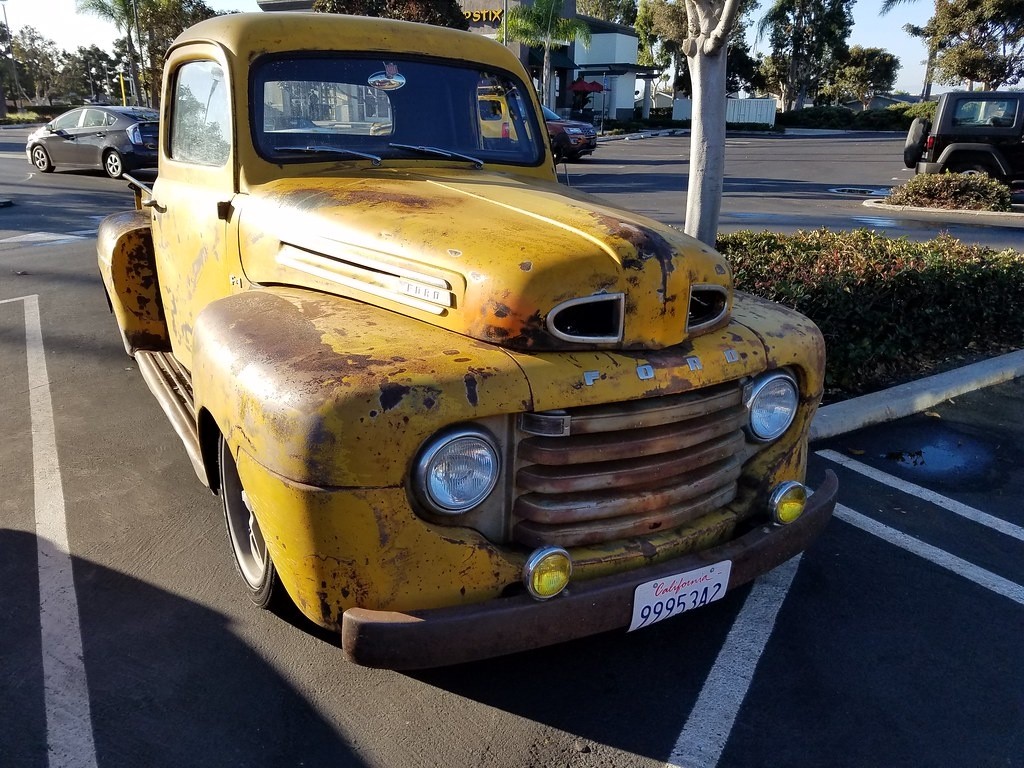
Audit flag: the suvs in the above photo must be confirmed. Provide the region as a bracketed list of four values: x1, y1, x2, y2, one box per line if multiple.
[902, 87, 1024, 186]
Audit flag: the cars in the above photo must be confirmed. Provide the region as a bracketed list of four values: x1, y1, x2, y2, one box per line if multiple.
[542, 104, 598, 164]
[94, 9, 841, 667]
[24, 105, 160, 180]
[369, 84, 532, 143]
[264, 113, 339, 134]
